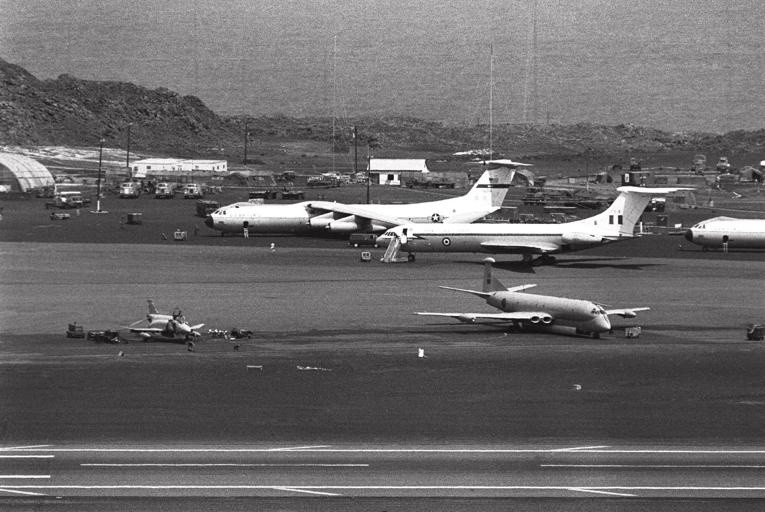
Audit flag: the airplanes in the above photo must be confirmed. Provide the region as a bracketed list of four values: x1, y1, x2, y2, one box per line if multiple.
[120, 298, 205, 343]
[411, 257, 651, 340]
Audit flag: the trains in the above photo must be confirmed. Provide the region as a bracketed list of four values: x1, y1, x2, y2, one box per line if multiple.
[49, 183, 102, 196]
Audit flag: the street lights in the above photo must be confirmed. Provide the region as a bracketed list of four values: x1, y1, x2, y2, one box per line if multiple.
[95, 138, 106, 211]
[127, 122, 133, 168]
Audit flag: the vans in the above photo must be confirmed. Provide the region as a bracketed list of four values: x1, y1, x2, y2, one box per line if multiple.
[45, 191, 84, 209]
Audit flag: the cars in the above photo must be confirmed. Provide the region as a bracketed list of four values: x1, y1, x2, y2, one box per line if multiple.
[155, 183, 175, 199]
[184, 184, 204, 199]
[120, 182, 140, 198]
